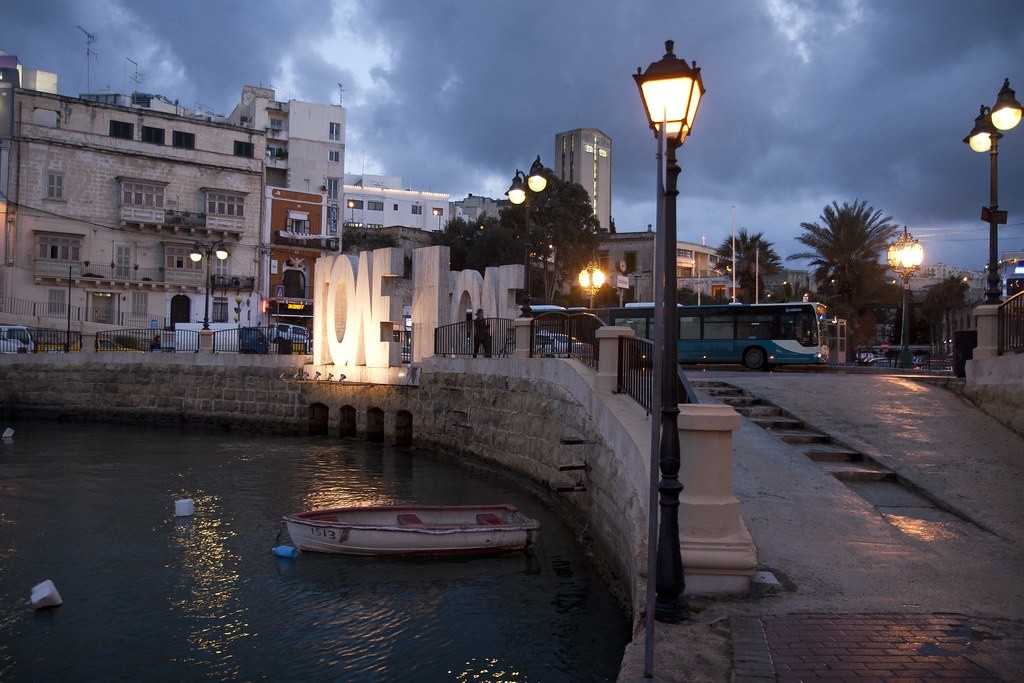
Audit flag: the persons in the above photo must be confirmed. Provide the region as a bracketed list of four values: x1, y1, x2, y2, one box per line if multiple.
[473, 309, 490, 357]
[151, 335, 161, 350]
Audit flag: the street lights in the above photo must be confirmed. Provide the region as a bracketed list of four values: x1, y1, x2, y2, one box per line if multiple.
[434, 211, 440, 230]
[887, 226, 924, 369]
[349, 202, 354, 222]
[579, 261, 605, 309]
[632, 39, 705, 624]
[963, 78, 1024, 304]
[504, 155, 547, 317]
[190, 240, 228, 330]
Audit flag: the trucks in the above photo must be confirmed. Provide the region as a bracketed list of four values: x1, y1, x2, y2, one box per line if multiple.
[175, 322, 270, 355]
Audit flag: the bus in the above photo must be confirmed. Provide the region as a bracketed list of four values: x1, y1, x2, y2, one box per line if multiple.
[610, 302, 830, 370]
[467, 305, 587, 336]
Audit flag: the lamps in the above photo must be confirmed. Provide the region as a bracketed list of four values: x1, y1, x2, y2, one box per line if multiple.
[303, 372, 309, 378]
[327, 373, 333, 381]
[340, 374, 346, 381]
[314, 372, 321, 379]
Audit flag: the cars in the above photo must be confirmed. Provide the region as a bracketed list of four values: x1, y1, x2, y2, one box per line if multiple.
[70, 337, 136, 351]
[270, 323, 310, 344]
[0, 326, 34, 354]
[534, 330, 594, 359]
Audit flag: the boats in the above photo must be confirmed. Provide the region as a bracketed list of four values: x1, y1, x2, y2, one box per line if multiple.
[282, 505, 542, 558]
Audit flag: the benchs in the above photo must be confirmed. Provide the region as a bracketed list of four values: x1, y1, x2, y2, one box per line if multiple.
[317, 517, 339, 521]
[476, 514, 507, 525]
[398, 514, 425, 525]
[498, 335, 556, 357]
